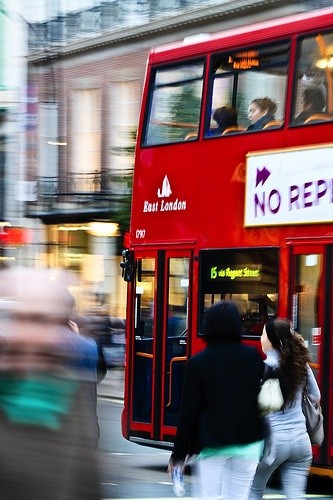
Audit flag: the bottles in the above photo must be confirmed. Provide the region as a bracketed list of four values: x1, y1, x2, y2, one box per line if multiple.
[172, 464, 185, 497]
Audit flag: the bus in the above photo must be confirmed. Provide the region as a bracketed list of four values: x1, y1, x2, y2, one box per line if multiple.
[119, 3, 333, 496]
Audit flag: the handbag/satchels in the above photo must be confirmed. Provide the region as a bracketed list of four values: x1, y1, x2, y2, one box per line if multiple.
[304, 383, 324, 446]
